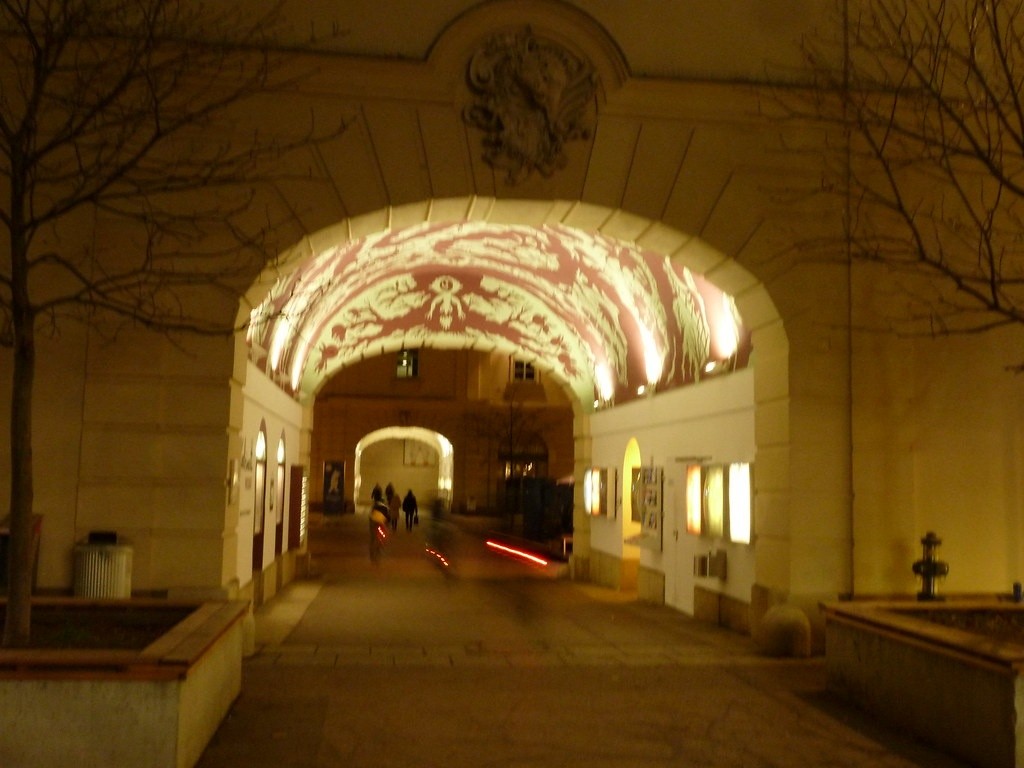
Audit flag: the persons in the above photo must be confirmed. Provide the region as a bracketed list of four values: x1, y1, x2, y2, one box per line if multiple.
[385, 481, 395, 505]
[371, 482, 382, 505]
[402, 490, 418, 533]
[368, 498, 391, 566]
[388, 491, 401, 532]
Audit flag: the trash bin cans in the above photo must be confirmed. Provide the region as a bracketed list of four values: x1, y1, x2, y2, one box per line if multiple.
[72, 543, 134, 598]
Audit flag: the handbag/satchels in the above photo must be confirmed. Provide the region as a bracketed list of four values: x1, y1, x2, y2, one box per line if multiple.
[414, 513, 419, 525]
